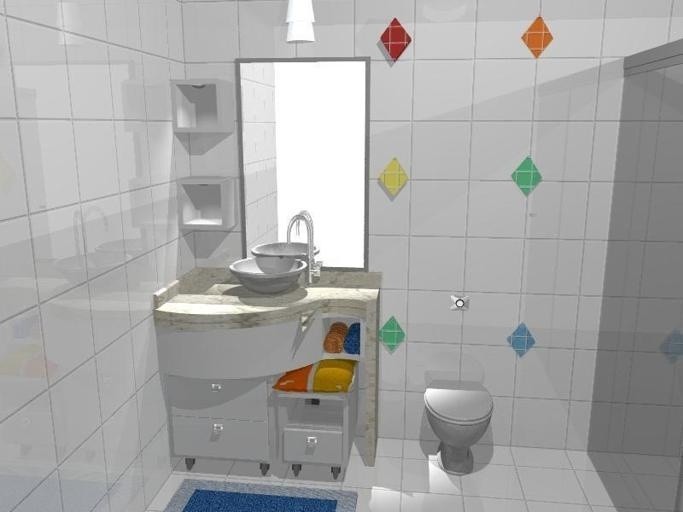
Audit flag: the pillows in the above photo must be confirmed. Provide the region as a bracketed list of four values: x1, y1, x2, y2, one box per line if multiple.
[272, 359, 357, 393]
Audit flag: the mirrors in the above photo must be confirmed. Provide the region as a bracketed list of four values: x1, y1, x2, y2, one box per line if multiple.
[233, 55, 370, 273]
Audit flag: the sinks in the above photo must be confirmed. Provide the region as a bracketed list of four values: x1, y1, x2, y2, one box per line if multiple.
[252, 241, 319, 256]
[229, 256, 308, 294]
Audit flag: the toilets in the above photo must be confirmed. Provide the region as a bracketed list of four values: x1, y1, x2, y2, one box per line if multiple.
[423, 381, 493, 473]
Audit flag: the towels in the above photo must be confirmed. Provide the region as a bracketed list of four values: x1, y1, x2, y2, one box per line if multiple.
[344, 322, 360, 354]
[323, 320, 348, 353]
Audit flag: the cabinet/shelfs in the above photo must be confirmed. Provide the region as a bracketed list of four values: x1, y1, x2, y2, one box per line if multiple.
[158, 314, 362, 480]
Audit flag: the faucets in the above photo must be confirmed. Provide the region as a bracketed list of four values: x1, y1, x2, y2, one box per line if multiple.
[286, 216, 316, 284]
[296, 211, 312, 237]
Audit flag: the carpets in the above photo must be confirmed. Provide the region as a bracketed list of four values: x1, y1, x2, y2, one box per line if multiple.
[164, 478, 358, 512]
